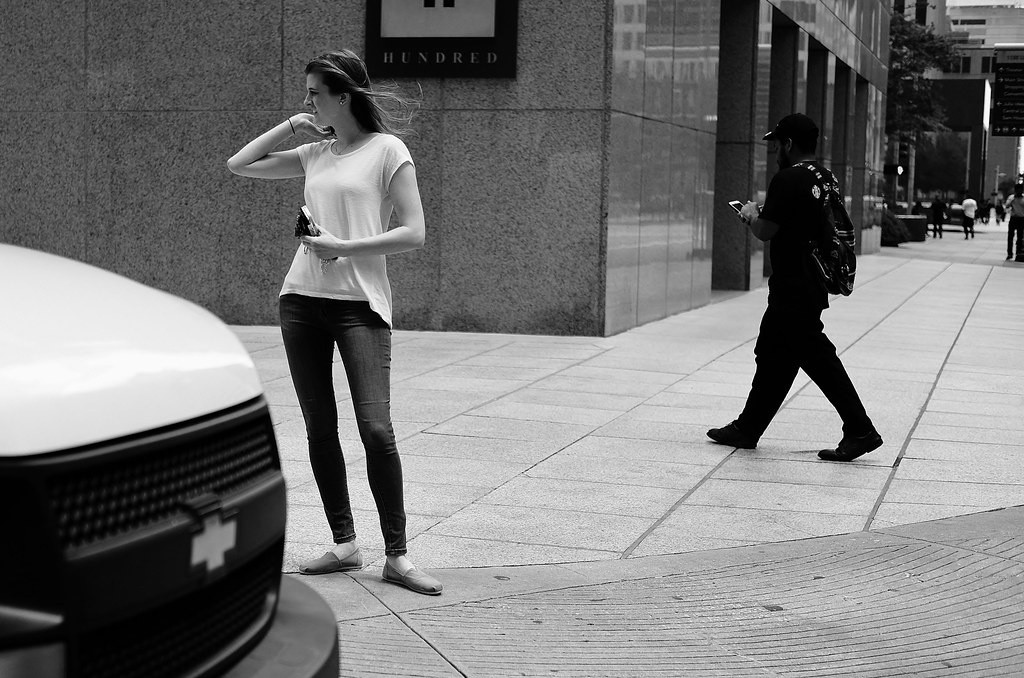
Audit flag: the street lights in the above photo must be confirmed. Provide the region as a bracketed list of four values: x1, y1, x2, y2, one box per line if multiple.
[995, 165, 1006, 209]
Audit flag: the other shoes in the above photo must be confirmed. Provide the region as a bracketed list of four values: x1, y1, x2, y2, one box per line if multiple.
[382, 557, 442, 595]
[818, 428, 882, 461]
[1006, 255, 1013, 260]
[298, 548, 362, 574]
[707, 420, 757, 449]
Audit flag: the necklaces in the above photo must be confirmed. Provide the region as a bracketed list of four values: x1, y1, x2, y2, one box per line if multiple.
[336, 129, 361, 154]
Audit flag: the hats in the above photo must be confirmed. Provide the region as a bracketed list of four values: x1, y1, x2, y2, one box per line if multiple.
[762, 112, 820, 145]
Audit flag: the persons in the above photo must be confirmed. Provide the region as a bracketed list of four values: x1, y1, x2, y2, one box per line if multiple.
[1004, 183, 1024, 263]
[226, 47, 445, 598]
[705, 110, 886, 461]
[912, 188, 1005, 240]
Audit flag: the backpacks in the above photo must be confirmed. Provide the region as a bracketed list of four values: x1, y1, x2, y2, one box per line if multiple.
[793, 161, 856, 297]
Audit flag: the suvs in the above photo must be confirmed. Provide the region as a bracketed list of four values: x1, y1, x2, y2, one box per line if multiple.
[0, 246, 339, 678]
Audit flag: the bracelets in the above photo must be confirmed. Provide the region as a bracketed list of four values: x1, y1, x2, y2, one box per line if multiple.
[287, 117, 297, 136]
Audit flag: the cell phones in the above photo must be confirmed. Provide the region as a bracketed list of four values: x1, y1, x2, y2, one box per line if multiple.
[302, 205, 320, 236]
[729, 200, 744, 216]
